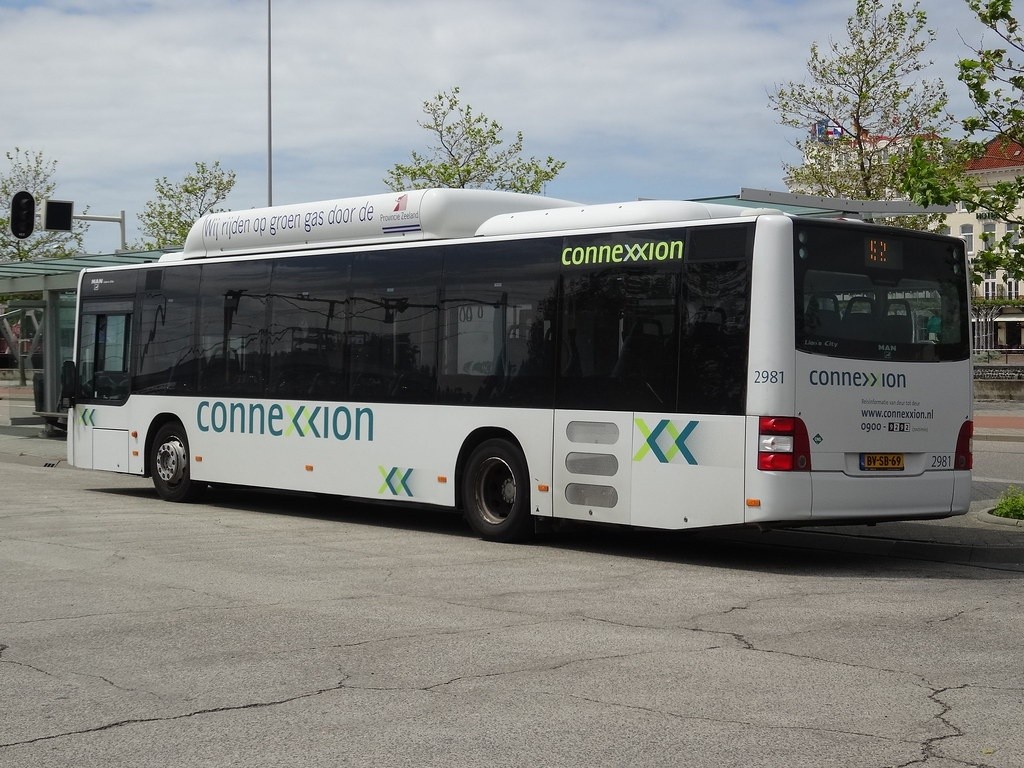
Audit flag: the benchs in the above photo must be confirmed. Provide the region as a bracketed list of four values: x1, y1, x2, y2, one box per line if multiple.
[32, 392, 68, 436]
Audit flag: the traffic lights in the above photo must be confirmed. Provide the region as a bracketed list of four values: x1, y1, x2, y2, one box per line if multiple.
[43, 197, 74, 232]
[10, 188, 36, 238]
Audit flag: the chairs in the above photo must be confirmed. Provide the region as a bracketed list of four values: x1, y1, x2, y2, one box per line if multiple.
[173, 293, 915, 402]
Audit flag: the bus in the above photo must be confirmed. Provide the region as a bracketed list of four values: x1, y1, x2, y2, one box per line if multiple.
[56, 174, 976, 539]
